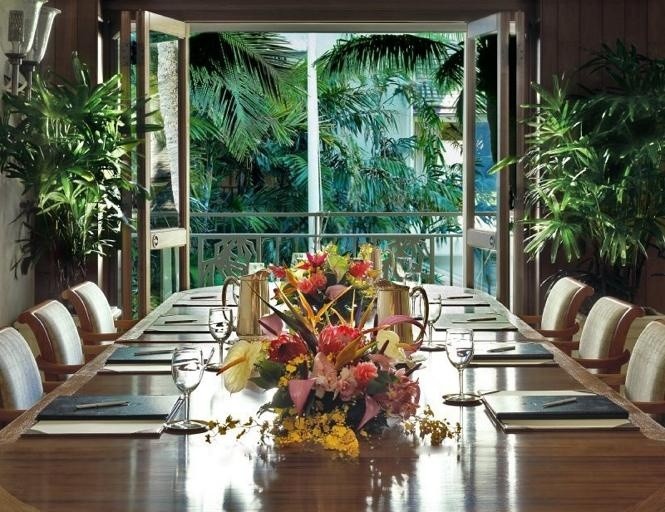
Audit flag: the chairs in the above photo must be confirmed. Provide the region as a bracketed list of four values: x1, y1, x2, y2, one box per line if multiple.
[0, 277, 141, 432]
[519, 277, 664, 424]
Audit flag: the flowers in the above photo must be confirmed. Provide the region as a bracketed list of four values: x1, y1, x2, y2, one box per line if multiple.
[201, 243, 464, 462]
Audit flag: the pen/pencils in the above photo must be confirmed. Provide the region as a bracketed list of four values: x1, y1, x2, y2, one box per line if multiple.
[543, 398, 577, 408]
[75, 400, 129, 410]
[190, 296, 216, 299]
[447, 295, 473, 299]
[134, 350, 173, 355]
[487, 346, 515, 352]
[467, 318, 496, 321]
[165, 320, 198, 324]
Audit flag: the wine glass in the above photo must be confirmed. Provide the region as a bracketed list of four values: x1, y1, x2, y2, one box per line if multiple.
[207, 307, 236, 371]
[443, 326, 482, 404]
[394, 255, 423, 318]
[418, 292, 445, 351]
[162, 346, 212, 432]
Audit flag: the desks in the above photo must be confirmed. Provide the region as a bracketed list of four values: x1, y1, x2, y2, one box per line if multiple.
[1, 282, 664, 512]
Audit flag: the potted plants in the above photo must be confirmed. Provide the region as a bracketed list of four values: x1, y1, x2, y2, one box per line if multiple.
[0, 50, 164, 397]
[487, 38, 665, 396]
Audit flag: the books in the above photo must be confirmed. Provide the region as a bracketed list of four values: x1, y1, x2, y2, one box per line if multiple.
[18, 290, 242, 439]
[420, 292, 643, 432]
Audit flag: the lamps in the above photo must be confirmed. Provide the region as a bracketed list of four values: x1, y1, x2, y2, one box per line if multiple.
[0, 0, 63, 101]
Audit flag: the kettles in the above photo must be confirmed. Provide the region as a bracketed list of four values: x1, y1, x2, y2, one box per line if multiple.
[372, 280, 430, 359]
[221, 268, 272, 339]
